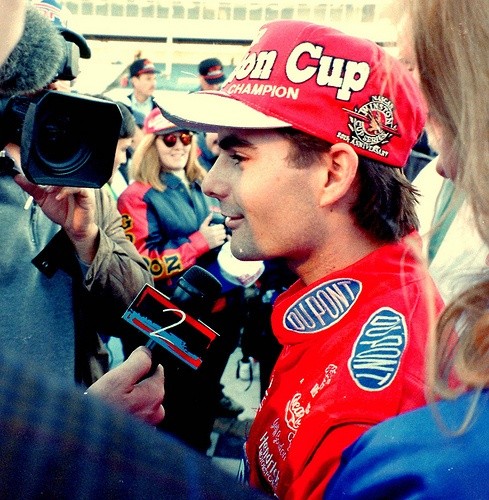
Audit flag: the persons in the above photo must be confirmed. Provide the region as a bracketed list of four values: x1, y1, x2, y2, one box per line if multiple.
[151, 19, 471, 500]
[0, 0, 489, 500]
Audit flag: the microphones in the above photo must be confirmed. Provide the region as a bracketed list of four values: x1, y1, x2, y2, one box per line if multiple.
[1, 3, 66, 96]
[134, 265, 223, 386]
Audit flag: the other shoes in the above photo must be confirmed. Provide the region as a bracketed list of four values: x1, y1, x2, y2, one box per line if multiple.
[218, 398, 244, 416]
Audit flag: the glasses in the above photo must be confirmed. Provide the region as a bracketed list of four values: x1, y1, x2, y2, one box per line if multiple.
[158, 132, 194, 148]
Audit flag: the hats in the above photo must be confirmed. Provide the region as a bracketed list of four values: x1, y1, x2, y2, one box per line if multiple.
[145, 108, 200, 136]
[129, 59, 160, 76]
[199, 57, 226, 85]
[152, 19, 427, 167]
